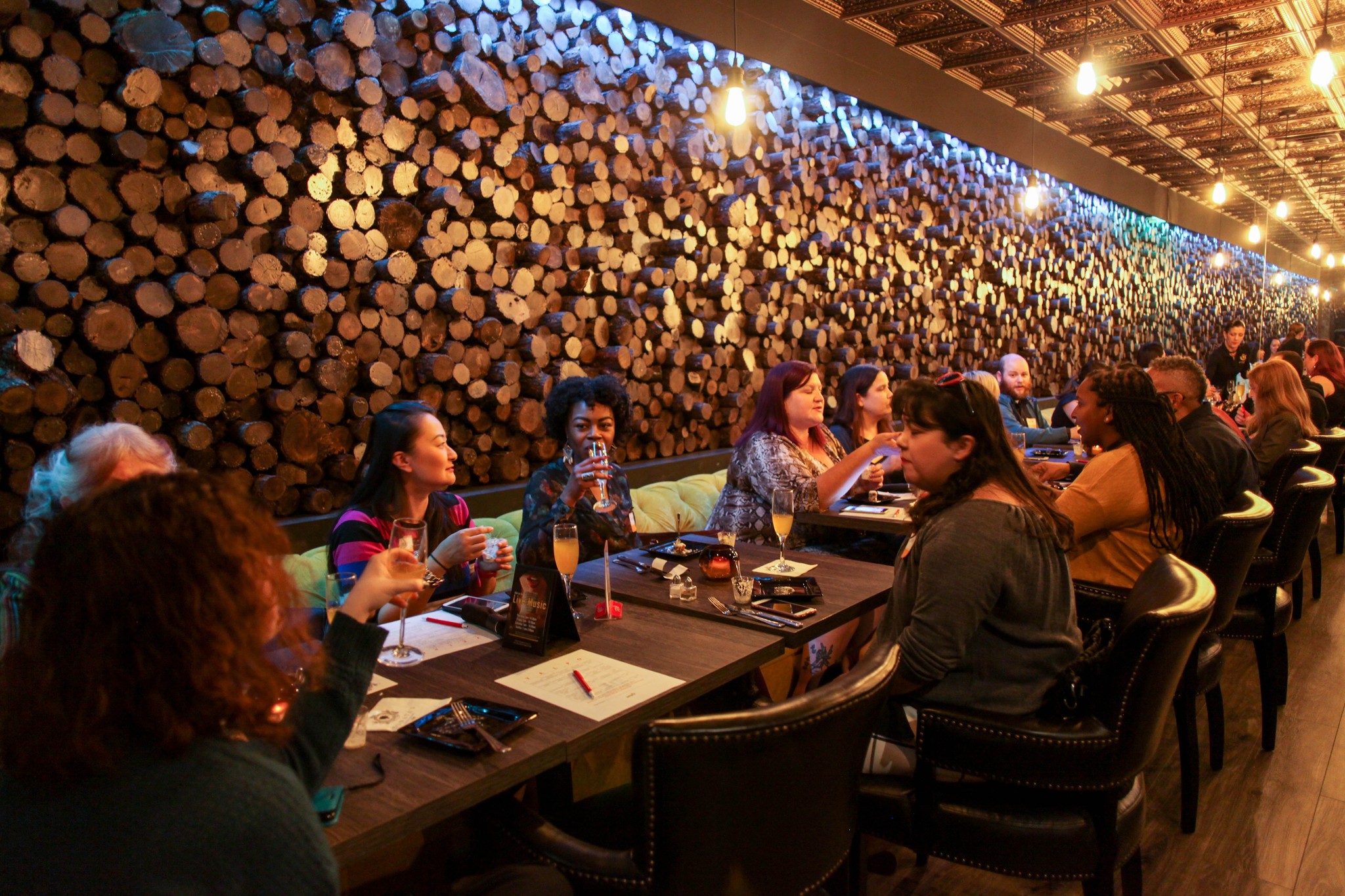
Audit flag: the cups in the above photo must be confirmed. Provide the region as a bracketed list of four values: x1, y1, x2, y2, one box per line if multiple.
[1091, 446, 1103, 458]
[1011, 433, 1025, 463]
[344, 705, 369, 749]
[326, 572, 357, 625]
[731, 576, 754, 604]
[1073, 443, 1084, 457]
[717, 531, 737, 547]
[482, 538, 498, 563]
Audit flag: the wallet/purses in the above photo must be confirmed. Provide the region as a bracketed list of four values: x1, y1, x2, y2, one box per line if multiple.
[309, 753, 386, 826]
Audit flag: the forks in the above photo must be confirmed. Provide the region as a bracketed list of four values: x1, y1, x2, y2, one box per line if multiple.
[707, 596, 785, 627]
[451, 702, 512, 753]
[612, 559, 653, 574]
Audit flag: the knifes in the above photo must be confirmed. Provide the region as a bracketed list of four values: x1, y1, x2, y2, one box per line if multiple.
[711, 603, 803, 629]
[617, 555, 673, 577]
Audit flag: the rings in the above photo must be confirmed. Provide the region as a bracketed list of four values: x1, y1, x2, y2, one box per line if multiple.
[580, 472, 594, 481]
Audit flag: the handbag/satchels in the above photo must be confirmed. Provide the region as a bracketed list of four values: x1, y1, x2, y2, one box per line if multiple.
[1037, 617, 1119, 727]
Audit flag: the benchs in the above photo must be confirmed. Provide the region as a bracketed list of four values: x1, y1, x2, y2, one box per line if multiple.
[276, 453, 744, 617]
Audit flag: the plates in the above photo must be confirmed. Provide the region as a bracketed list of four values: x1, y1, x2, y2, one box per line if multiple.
[840, 492, 901, 506]
[639, 539, 710, 557]
[1030, 449, 1068, 457]
[397, 696, 538, 757]
[753, 577, 823, 601]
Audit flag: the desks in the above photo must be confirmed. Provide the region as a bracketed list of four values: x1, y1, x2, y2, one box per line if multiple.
[800, 492, 917, 538]
[249, 538, 897, 890]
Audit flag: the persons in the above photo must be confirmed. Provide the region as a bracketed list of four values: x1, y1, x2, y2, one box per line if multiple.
[704, 320, 1345, 583]
[0, 424, 423, 896]
[863, 372, 1081, 877]
[512, 570, 548, 631]
[328, 401, 517, 629]
[515, 375, 659, 568]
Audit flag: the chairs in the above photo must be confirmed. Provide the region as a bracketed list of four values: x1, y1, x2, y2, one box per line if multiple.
[489, 641, 900, 896]
[862, 554, 1215, 896]
[1261, 427, 1345, 619]
[1173, 491, 1274, 829]
[1217, 465, 1336, 748]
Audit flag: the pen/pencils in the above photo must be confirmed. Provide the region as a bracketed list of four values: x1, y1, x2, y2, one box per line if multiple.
[573, 670, 595, 698]
[1075, 459, 1087, 463]
[426, 616, 467, 627]
[893, 509, 900, 517]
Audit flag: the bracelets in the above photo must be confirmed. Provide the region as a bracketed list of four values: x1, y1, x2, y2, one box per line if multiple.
[422, 554, 447, 587]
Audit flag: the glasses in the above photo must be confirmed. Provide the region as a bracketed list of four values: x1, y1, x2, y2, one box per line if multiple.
[936, 372, 983, 429]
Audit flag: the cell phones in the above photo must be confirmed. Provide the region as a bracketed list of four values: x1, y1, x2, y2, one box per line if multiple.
[749, 598, 817, 619]
[309, 785, 345, 827]
[442, 595, 510, 615]
[676, 534, 721, 545]
[839, 505, 889, 513]
[1023, 455, 1048, 461]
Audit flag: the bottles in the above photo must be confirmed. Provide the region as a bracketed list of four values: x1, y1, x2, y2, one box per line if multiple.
[669, 574, 684, 598]
[699, 544, 739, 582]
[680, 576, 697, 602]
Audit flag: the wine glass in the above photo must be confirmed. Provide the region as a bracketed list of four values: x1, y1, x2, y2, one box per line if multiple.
[769, 488, 796, 572]
[377, 518, 428, 667]
[553, 523, 584, 620]
[588, 441, 617, 513]
[1207, 380, 1245, 405]
